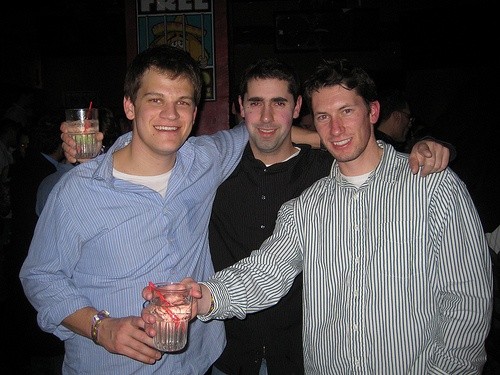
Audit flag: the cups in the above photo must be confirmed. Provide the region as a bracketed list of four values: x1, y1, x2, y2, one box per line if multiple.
[65, 108, 99, 163]
[151, 282, 192, 352]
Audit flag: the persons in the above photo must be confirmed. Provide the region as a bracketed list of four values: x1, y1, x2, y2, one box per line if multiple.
[19, 45, 327, 375]
[232, 93, 415, 153]
[61, 58, 449, 375]
[142, 65, 493, 375]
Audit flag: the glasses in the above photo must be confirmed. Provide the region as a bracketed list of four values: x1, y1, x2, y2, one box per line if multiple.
[393, 109, 415, 122]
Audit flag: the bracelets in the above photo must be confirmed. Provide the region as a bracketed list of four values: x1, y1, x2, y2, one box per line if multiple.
[204, 294, 214, 316]
[92, 310, 111, 344]
